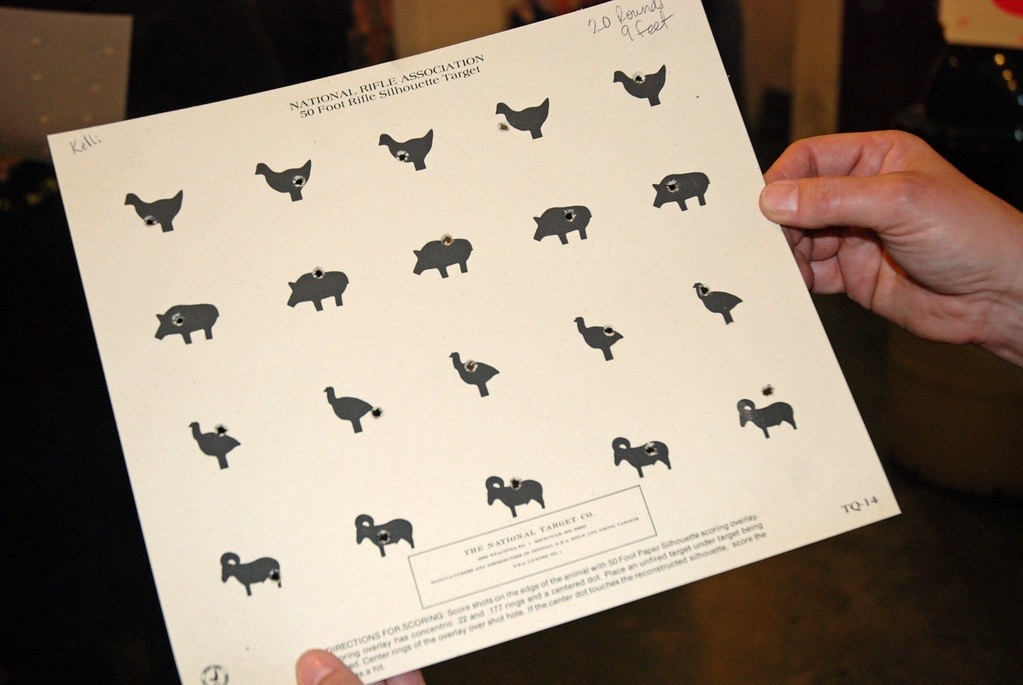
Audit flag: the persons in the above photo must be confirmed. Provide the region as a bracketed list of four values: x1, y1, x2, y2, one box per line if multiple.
[294, 129, 1022, 685]
